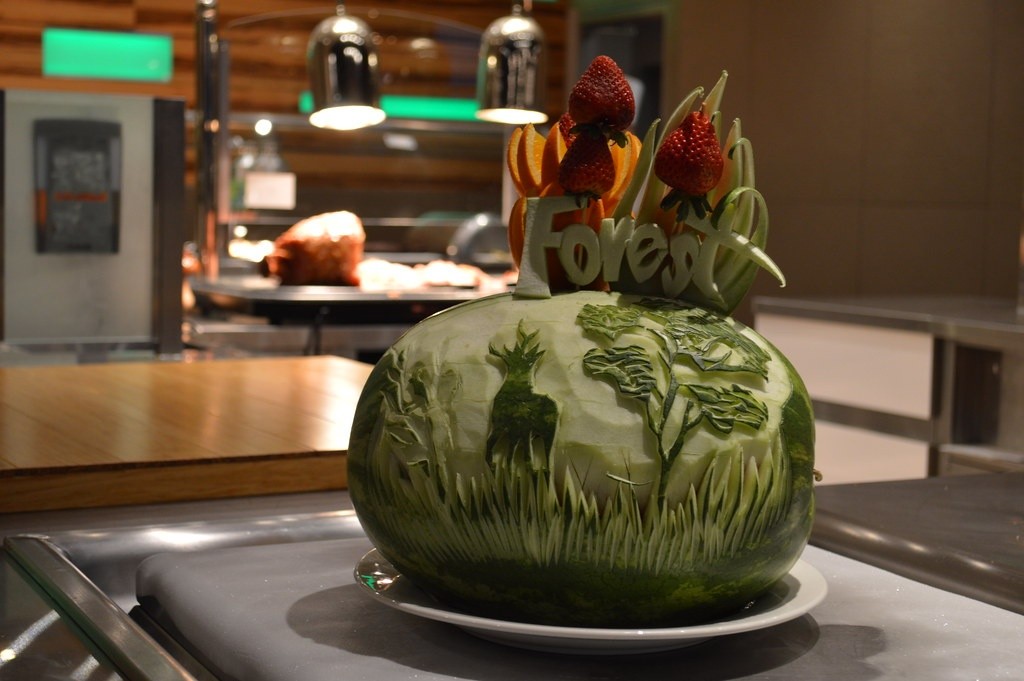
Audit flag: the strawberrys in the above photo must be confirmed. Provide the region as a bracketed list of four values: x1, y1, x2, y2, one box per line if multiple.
[558, 55, 724, 219]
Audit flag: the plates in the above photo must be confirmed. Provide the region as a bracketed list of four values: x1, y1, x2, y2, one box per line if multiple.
[353, 545, 828, 654]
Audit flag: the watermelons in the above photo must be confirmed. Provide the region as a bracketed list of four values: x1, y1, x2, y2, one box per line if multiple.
[346, 288, 817, 618]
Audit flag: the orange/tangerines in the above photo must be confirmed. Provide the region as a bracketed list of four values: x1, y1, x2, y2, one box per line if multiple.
[506, 123, 639, 278]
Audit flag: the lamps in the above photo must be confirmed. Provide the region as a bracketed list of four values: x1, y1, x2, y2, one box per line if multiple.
[308, 11, 390, 134]
[474, 3, 554, 127]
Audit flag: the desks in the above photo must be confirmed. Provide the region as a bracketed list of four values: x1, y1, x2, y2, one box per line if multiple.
[747, 296, 1024, 486]
[1, 352, 376, 518]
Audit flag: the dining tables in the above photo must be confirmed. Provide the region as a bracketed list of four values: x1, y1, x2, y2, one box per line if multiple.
[3, 473, 1022, 681]
